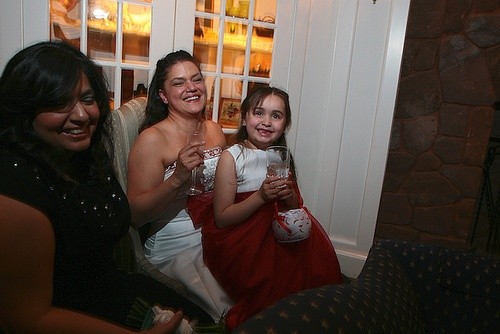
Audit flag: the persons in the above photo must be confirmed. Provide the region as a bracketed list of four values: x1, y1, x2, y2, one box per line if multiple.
[187, 86, 343, 333]
[126, 50, 239, 324]
[0, 40, 197, 334]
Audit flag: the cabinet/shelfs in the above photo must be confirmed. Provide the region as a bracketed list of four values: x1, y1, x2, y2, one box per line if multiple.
[50, 0, 278, 135]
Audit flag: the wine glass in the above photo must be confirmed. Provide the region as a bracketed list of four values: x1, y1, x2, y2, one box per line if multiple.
[183, 131, 204, 196]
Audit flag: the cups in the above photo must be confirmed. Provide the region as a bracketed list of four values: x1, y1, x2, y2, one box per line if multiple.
[265, 145, 290, 190]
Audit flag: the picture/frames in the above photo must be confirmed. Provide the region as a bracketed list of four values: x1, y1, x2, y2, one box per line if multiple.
[220, 98, 240, 128]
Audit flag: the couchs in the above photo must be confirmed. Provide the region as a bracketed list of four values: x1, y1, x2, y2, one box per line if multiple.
[231, 239, 500, 334]
[106, 97, 228, 325]
